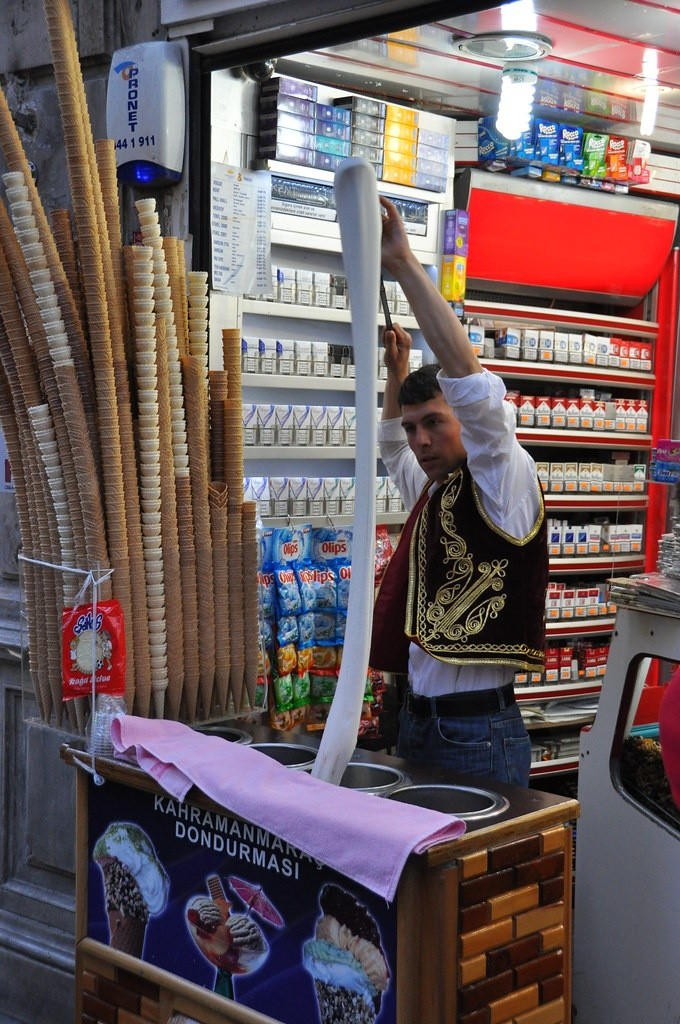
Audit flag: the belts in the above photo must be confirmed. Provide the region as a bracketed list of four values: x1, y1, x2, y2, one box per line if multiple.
[405, 687, 517, 717]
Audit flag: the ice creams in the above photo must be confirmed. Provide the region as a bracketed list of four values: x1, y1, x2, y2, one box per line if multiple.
[303, 883, 392, 1024]
[184, 874, 268, 976]
[92, 822, 171, 961]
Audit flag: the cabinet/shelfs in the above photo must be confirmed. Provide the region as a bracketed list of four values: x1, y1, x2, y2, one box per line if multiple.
[191, 60, 454, 528]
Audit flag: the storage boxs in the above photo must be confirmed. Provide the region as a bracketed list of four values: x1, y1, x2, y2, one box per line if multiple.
[240, 76, 653, 763]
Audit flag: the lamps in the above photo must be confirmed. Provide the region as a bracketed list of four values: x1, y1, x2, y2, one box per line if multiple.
[452, 0, 553, 61]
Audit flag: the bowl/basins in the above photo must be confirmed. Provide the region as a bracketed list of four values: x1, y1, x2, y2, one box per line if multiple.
[91, 695, 126, 760]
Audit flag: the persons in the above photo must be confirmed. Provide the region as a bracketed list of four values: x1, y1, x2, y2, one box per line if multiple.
[369, 194, 547, 792]
